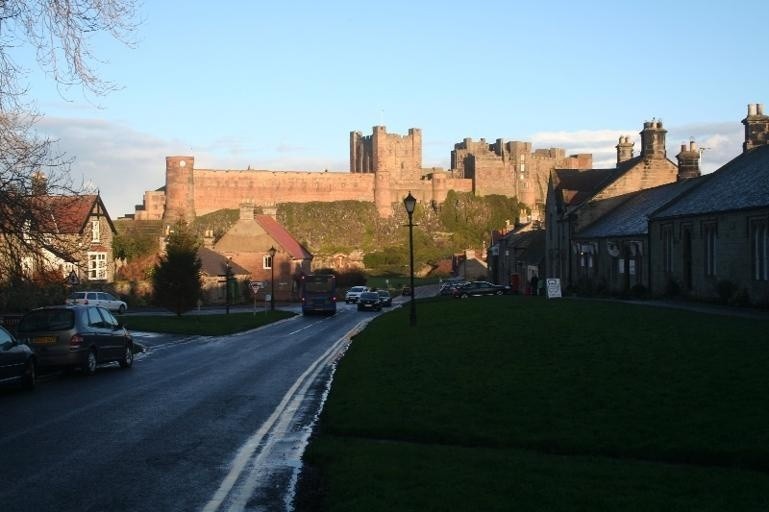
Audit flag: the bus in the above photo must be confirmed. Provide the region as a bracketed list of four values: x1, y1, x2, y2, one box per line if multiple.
[302, 274, 337, 316]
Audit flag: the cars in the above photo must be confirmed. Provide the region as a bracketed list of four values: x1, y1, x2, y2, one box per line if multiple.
[378, 291, 393, 306]
[0, 325, 37, 392]
[357, 292, 382, 311]
[345, 286, 370, 303]
[66, 291, 128, 314]
[402, 287, 411, 296]
[440, 278, 513, 299]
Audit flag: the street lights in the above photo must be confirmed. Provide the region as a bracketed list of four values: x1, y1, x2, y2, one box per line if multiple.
[404, 191, 418, 326]
[225, 259, 229, 314]
[268, 245, 276, 311]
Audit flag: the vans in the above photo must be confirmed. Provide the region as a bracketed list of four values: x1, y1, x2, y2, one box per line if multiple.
[17, 304, 134, 378]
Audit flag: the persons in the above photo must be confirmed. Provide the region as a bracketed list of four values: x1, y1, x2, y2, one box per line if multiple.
[530, 273, 539, 297]
